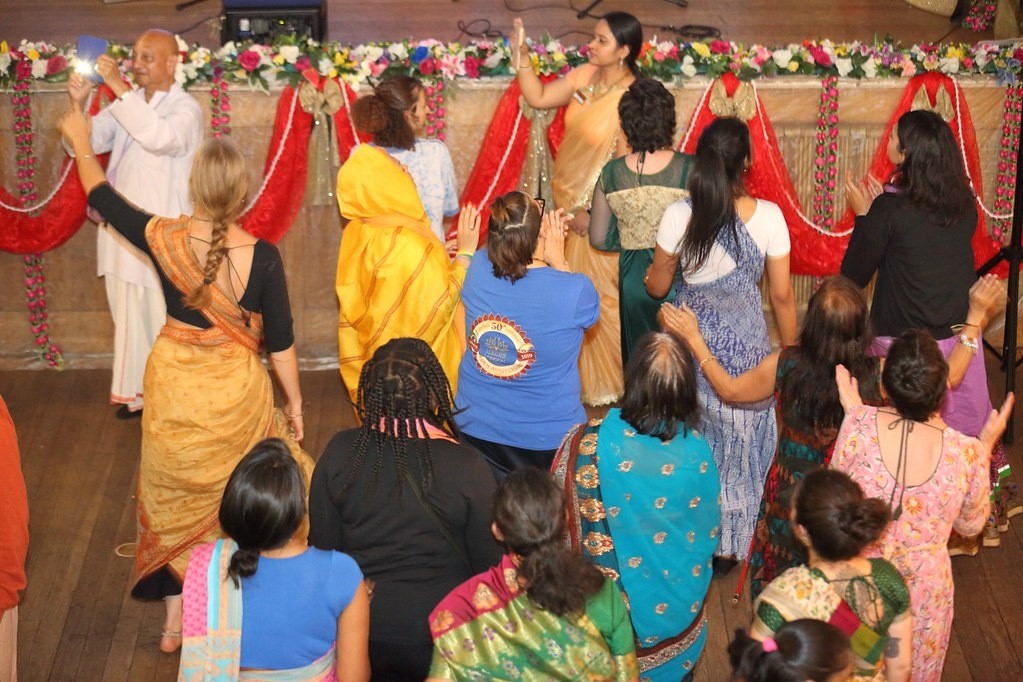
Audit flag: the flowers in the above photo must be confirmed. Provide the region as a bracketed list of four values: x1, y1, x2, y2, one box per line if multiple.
[0, 31, 1023, 373]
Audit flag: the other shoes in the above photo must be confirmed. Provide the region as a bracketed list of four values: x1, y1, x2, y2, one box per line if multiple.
[116, 404, 143, 419]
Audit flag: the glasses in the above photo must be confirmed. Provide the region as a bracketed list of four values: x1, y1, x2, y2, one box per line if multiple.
[534, 197, 546, 221]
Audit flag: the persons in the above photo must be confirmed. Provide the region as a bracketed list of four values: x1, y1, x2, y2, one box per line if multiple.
[424, 465, 639, 682]
[544, 330, 722, 682]
[727, 467, 912, 682]
[178, 438, 371, 682]
[62, 28, 204, 419]
[659, 275, 1005, 626]
[840, 108, 1023, 555]
[307, 337, 510, 682]
[58, 100, 315, 654]
[830, 328, 1013, 682]
[0, 396, 29, 682]
[337, 9, 795, 570]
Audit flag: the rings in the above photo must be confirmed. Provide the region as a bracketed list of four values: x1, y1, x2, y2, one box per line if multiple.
[469, 225, 474, 230]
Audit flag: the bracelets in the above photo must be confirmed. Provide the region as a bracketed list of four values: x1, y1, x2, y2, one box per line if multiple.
[949, 315, 985, 354]
[519, 60, 534, 71]
[76, 155, 96, 161]
[645, 276, 650, 282]
[118, 88, 131, 101]
[698, 355, 719, 371]
[282, 408, 306, 418]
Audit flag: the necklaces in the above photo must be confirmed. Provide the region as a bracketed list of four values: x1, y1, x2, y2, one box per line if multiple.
[532, 255, 546, 265]
[585, 71, 629, 103]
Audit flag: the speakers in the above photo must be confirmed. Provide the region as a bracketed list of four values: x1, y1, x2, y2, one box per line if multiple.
[222, 0, 327, 48]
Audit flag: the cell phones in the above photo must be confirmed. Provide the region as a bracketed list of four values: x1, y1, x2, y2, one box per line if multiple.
[75, 36, 109, 81]
